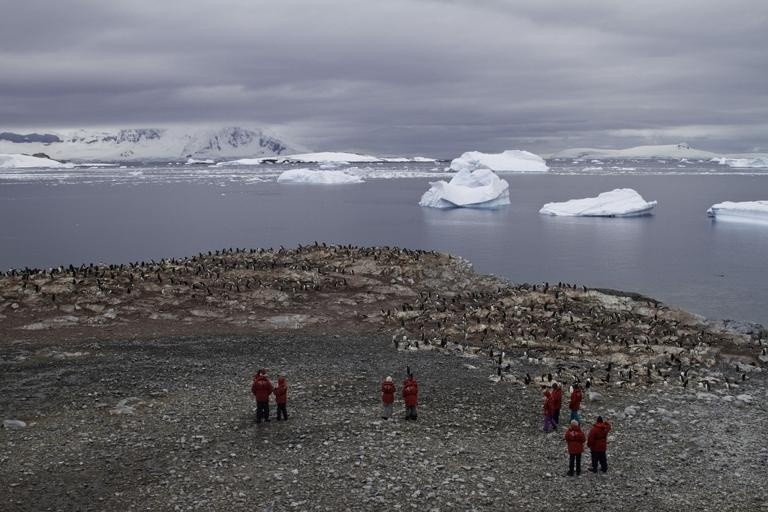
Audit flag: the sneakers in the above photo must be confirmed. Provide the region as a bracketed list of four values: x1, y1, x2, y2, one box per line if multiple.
[276, 415, 289, 421]
[567, 471, 581, 476]
[587, 467, 607, 473]
[405, 415, 418, 420]
[256, 418, 272, 424]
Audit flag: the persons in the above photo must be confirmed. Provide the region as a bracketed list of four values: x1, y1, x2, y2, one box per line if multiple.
[564, 419, 586, 477]
[568, 384, 583, 428]
[253, 368, 266, 417]
[401, 378, 418, 421]
[381, 376, 396, 420]
[273, 375, 289, 420]
[403, 373, 419, 408]
[251, 372, 273, 423]
[586, 415, 612, 473]
[550, 382, 562, 427]
[542, 389, 558, 432]
